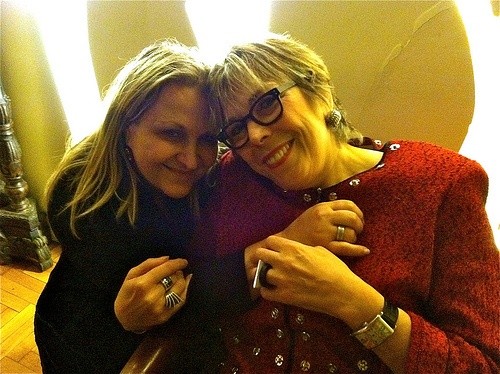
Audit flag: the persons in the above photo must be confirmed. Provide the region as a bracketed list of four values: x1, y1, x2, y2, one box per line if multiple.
[34, 38, 223, 374]
[189, 29, 500, 374]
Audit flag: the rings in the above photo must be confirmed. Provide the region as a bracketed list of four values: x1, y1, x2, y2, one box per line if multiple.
[253, 260, 274, 289]
[161, 276, 172, 289]
[336, 225, 344, 241]
[165, 291, 181, 307]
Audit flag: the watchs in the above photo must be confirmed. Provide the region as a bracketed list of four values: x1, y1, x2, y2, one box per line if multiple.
[352, 297, 399, 350]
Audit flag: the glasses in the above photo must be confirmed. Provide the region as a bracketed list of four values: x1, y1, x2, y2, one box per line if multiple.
[216, 80, 297, 148]
